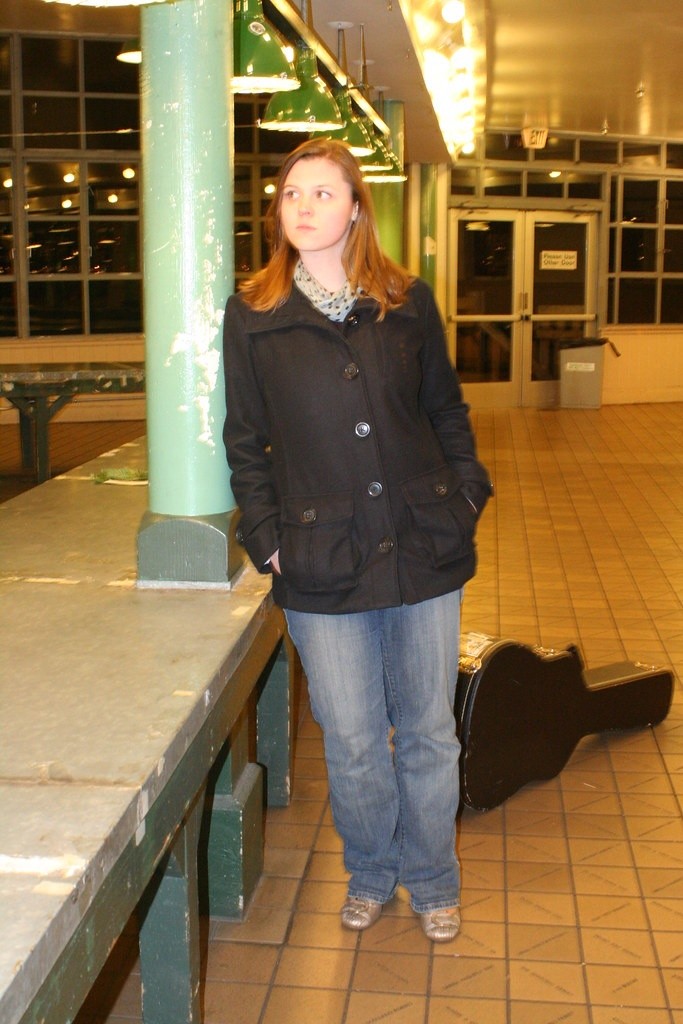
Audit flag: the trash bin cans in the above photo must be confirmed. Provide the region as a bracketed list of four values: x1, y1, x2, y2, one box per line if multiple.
[555, 336, 606, 409]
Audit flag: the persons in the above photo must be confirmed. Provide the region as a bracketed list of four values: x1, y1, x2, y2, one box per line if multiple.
[221, 137, 494, 943]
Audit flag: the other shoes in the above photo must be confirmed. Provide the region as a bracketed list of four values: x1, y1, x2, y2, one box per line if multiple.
[420, 906, 460, 942]
[340, 894, 381, 930]
